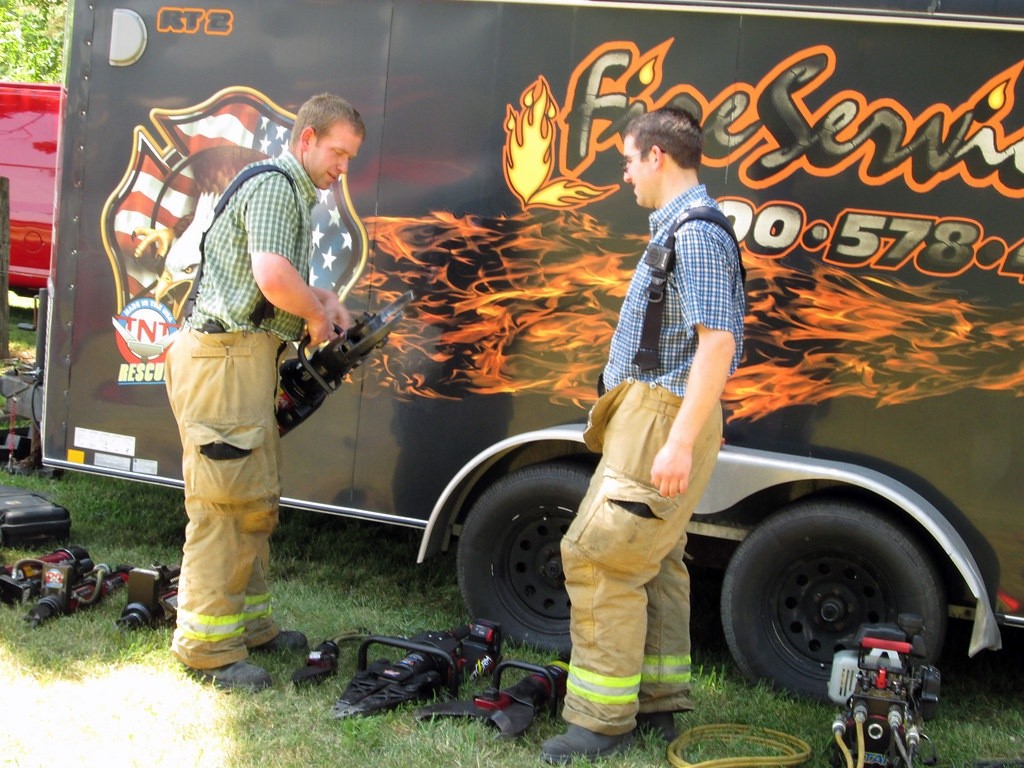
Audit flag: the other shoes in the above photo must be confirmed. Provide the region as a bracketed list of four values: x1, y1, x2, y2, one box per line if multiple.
[182, 660, 272, 693]
[633, 711, 676, 743]
[542, 722, 639, 762]
[247, 630, 308, 653]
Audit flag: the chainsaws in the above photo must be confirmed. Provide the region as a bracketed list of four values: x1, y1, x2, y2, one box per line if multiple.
[276, 289, 417, 441]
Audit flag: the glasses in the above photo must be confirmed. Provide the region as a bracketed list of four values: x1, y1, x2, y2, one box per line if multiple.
[617, 147, 666, 173]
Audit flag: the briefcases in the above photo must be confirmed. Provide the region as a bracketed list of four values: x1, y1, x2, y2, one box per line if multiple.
[0, 493, 71, 548]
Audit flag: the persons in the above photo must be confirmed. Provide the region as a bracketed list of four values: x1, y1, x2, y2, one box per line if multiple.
[533, 110, 747, 766]
[166, 91, 369, 702]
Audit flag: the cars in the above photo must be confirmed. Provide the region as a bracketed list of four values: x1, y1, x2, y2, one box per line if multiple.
[0, 82, 63, 298]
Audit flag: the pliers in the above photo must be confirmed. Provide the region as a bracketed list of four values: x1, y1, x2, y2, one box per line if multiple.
[329, 619, 503, 726]
